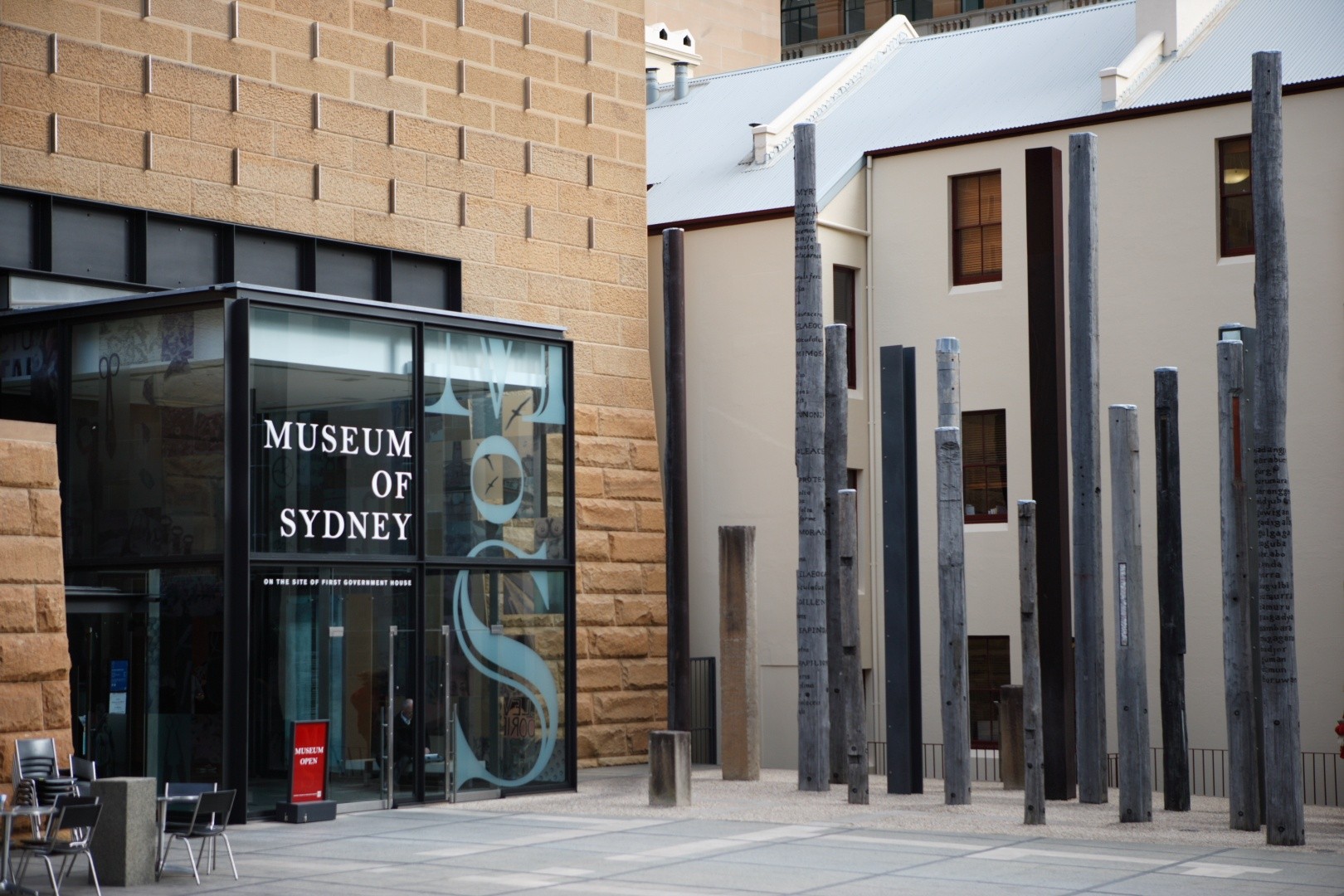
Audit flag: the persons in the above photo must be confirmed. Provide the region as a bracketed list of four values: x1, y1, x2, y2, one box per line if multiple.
[187, 663, 218, 706]
[393, 699, 431, 790]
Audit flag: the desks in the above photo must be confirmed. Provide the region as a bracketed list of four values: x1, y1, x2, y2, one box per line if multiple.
[1, 805, 58, 882]
[156, 794, 200, 874]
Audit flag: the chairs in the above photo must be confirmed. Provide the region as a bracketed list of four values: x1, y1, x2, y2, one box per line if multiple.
[0, 736, 239, 896]
